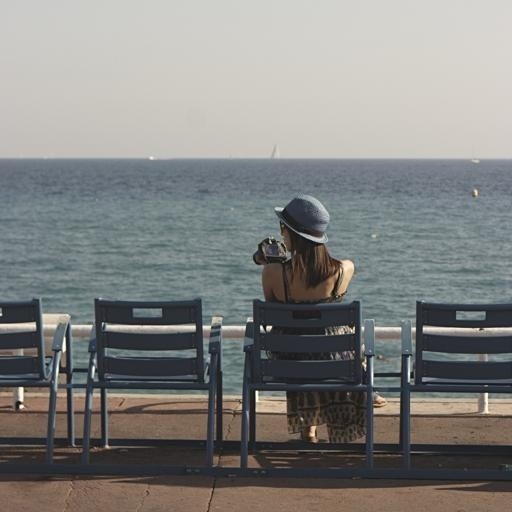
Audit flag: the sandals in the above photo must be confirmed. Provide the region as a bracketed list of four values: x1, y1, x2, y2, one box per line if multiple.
[373, 393, 386, 407]
[302, 430, 319, 444]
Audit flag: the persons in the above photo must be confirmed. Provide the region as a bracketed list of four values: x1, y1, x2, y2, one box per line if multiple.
[262, 195, 387, 443]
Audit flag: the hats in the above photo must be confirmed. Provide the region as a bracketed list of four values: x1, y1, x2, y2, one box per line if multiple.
[274, 194, 330, 244]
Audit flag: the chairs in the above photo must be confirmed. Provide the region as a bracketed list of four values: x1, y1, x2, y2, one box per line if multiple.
[396, 301, 512, 482]
[83, 297, 226, 477]
[0, 298, 77, 473]
[239, 300, 380, 476]
